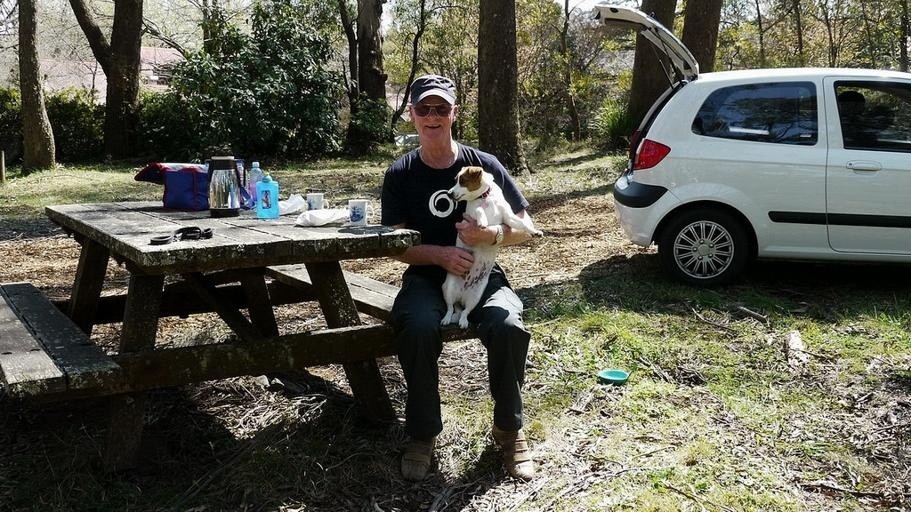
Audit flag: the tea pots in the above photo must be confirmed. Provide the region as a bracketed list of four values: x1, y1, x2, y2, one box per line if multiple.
[204, 154, 247, 218]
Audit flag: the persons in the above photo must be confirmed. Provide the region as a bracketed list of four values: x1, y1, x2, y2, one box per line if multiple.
[838, 91, 877, 148]
[382, 74, 535, 481]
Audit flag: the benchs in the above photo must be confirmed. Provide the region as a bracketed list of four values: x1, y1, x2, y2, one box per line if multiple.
[266, 256, 485, 355]
[0, 276, 123, 418]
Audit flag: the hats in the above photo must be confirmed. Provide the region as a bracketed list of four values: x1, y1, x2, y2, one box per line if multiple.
[409, 74, 457, 105]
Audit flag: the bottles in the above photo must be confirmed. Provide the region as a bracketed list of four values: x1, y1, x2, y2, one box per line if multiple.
[235, 160, 244, 187]
[249, 160, 264, 202]
[254, 175, 280, 219]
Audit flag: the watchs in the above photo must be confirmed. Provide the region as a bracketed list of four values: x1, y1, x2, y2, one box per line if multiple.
[491, 225, 504, 246]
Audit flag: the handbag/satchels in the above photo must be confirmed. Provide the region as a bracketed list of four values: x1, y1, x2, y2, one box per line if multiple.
[133, 161, 255, 212]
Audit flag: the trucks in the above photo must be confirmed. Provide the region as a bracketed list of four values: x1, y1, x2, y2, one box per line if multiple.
[139, 46, 184, 85]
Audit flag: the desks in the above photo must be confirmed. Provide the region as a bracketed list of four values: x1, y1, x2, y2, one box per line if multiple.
[44, 198, 423, 465]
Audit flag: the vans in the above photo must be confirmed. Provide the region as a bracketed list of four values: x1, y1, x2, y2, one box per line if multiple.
[591, 4, 911, 288]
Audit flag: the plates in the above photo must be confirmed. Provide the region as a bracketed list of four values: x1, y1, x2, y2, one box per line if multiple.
[595, 369, 630, 383]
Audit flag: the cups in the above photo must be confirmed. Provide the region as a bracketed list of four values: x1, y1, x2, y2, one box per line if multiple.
[348, 199, 375, 226]
[305, 193, 330, 209]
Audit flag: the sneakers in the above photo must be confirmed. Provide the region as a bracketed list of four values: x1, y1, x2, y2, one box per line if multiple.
[490, 421, 535, 483]
[399, 429, 437, 482]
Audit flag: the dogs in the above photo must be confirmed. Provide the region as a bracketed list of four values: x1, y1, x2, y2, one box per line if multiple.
[439, 165, 544, 331]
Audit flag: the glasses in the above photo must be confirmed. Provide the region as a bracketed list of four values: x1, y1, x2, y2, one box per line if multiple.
[411, 103, 454, 118]
